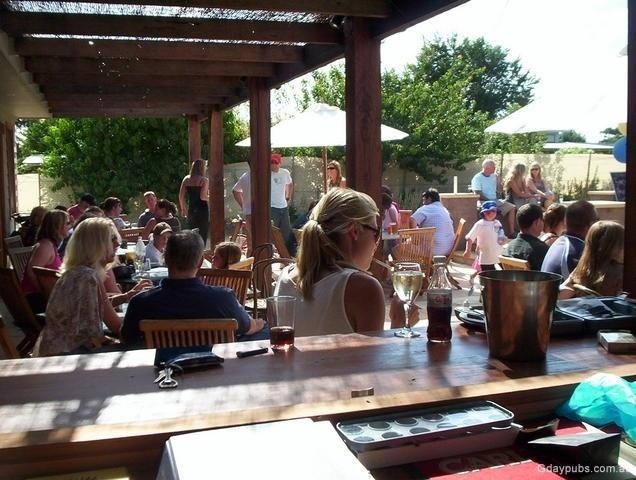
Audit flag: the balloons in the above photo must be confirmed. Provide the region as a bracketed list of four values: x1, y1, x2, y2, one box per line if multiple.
[618, 123, 627, 135]
[613, 137, 626, 163]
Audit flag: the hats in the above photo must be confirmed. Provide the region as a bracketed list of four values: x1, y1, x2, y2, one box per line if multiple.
[153, 222, 173, 236]
[80, 193, 96, 206]
[271, 153, 282, 164]
[479, 200, 498, 214]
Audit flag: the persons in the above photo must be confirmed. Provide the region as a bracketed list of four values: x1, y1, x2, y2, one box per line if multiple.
[471, 159, 515, 239]
[269, 154, 292, 245]
[324, 160, 346, 192]
[231, 159, 253, 259]
[179, 158, 209, 245]
[504, 162, 527, 202]
[525, 162, 553, 198]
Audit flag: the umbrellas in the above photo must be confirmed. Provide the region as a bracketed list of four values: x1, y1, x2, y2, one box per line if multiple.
[234, 103, 414, 189]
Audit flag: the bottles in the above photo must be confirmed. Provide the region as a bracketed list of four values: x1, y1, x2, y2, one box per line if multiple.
[136, 236, 146, 257]
[426, 256, 454, 342]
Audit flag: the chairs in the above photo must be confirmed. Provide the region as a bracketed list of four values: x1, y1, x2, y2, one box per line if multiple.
[0, 208, 601, 360]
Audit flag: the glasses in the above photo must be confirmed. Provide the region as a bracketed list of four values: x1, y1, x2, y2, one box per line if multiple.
[358, 222, 384, 242]
[327, 166, 334, 171]
[531, 168, 540, 171]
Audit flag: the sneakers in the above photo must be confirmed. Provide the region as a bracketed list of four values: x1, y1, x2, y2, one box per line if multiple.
[507, 232, 517, 239]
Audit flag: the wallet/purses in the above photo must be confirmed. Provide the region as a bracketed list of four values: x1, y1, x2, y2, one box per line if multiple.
[151, 344, 227, 375]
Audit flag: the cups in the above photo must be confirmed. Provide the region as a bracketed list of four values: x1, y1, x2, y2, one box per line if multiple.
[477, 270, 563, 371]
[265, 295, 298, 351]
[388, 223, 398, 235]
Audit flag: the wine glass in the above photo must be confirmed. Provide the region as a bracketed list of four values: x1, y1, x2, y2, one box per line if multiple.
[391, 263, 423, 340]
